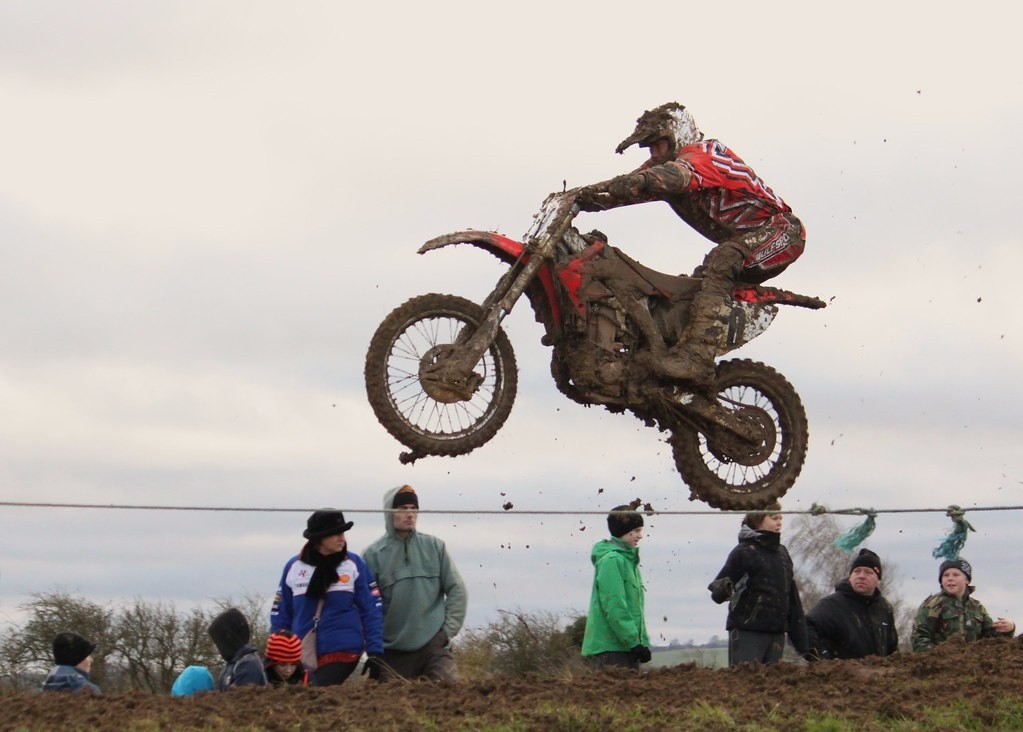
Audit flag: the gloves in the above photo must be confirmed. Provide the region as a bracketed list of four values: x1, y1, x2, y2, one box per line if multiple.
[631, 644, 651, 663]
[359, 656, 385, 679]
[607, 174, 647, 199]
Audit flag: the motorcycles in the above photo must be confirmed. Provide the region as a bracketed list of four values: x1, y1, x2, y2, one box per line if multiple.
[365, 184, 828, 512]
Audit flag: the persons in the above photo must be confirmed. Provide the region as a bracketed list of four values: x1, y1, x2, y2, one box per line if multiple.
[270, 507, 392, 686]
[358, 484, 467, 680]
[581, 506, 651, 676]
[808, 548, 900, 659]
[171, 608, 315, 697]
[575, 102, 808, 385]
[708, 502, 809, 669]
[43, 633, 102, 695]
[910, 558, 1016, 653]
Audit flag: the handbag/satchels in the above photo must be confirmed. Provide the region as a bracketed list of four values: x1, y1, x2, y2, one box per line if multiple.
[299, 631, 318, 671]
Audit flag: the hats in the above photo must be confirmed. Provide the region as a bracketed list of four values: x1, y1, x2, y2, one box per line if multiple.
[302, 508, 354, 540]
[264, 630, 302, 662]
[607, 505, 645, 538]
[851, 548, 883, 580]
[52, 632, 97, 665]
[394, 486, 418, 507]
[939, 558, 972, 583]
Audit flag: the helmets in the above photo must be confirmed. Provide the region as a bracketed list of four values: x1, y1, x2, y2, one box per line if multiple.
[616, 104, 699, 164]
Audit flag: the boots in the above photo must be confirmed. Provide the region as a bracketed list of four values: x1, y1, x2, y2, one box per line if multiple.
[649, 292, 730, 384]
